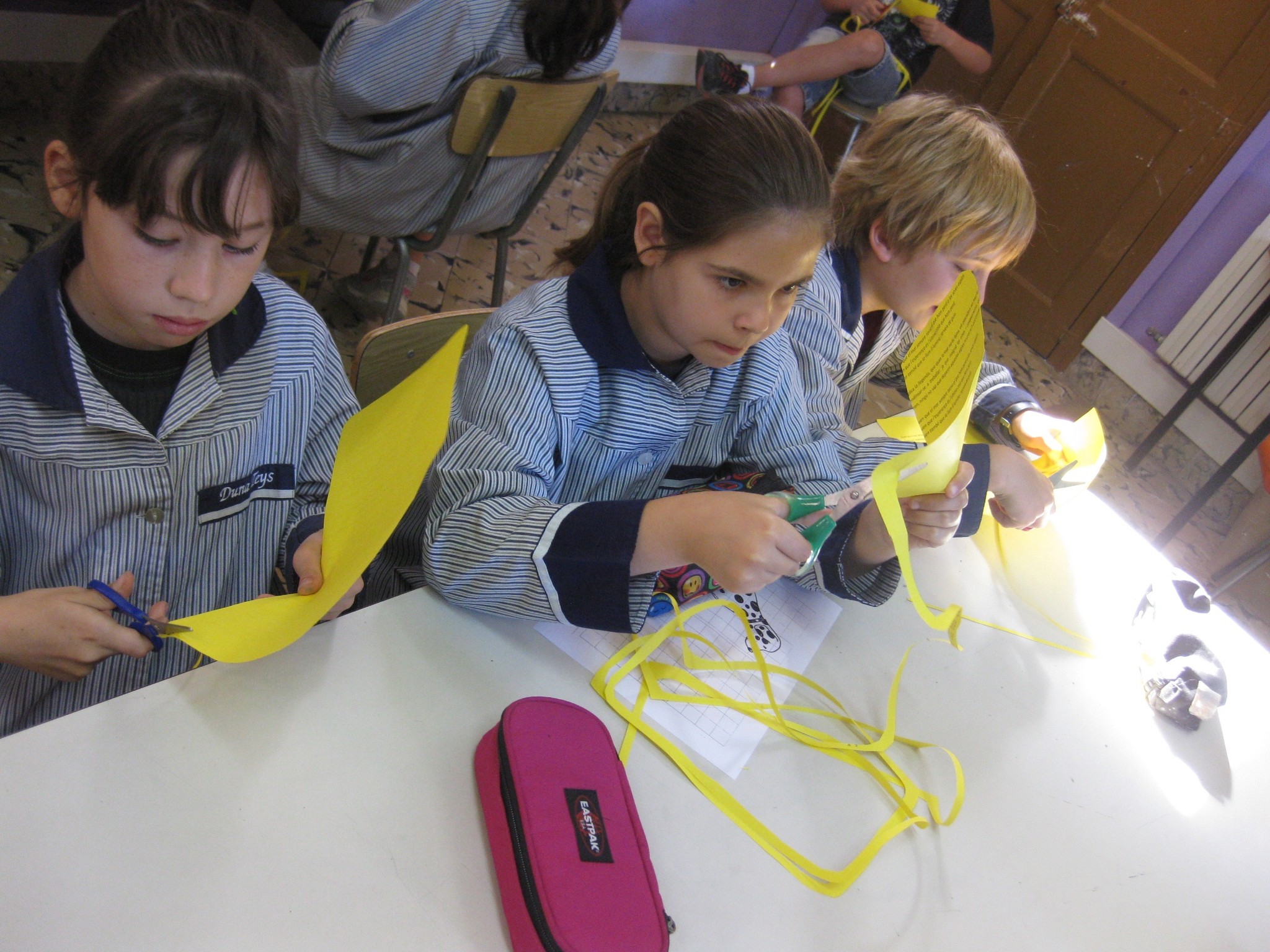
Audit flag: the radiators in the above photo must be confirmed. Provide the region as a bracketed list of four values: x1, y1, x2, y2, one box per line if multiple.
[1146, 211, 1268, 438]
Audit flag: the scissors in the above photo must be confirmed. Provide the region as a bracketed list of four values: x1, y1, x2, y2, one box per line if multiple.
[878, 2, 900, 20]
[994, 460, 1085, 516]
[87, 579, 194, 653]
[763, 458, 928, 578]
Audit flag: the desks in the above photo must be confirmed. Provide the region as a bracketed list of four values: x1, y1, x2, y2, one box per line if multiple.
[1, 391, 1267, 949]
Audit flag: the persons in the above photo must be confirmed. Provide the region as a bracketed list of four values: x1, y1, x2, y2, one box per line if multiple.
[785, 87, 1109, 538]
[366, 47, 975, 636]
[692, 0, 997, 118]
[275, 0, 624, 319]
[1, 0, 368, 737]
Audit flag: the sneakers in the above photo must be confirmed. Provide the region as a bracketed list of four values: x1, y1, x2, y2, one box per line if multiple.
[694, 49, 752, 100]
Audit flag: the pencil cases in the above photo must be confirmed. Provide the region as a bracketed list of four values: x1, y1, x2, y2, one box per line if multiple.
[472, 696, 677, 952]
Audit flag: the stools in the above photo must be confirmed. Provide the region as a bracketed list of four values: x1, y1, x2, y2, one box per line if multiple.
[809, 90, 884, 176]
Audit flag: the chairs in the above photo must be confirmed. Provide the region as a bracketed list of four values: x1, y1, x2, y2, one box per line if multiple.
[350, 70, 623, 323]
[352, 305, 501, 409]
